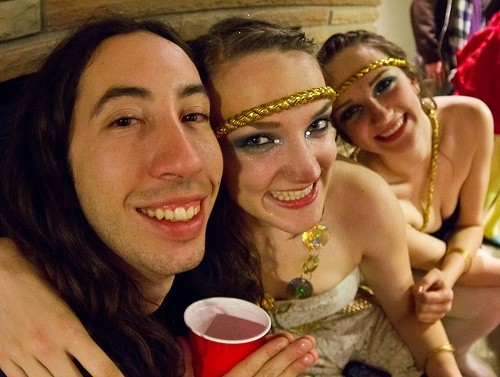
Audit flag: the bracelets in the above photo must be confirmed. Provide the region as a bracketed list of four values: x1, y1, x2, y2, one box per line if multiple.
[418, 344, 456, 369]
[442, 247, 473, 274]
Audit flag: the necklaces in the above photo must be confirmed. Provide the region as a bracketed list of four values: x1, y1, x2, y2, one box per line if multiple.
[250, 206, 331, 311]
[350, 100, 439, 231]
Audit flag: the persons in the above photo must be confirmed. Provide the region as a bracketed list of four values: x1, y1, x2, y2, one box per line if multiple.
[0, 16, 463, 377]
[315, 29, 500, 377]
[411, 0, 500, 257]
[0, 17, 318, 377]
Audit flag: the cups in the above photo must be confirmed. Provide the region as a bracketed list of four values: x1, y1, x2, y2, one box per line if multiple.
[182, 296, 274, 377]
